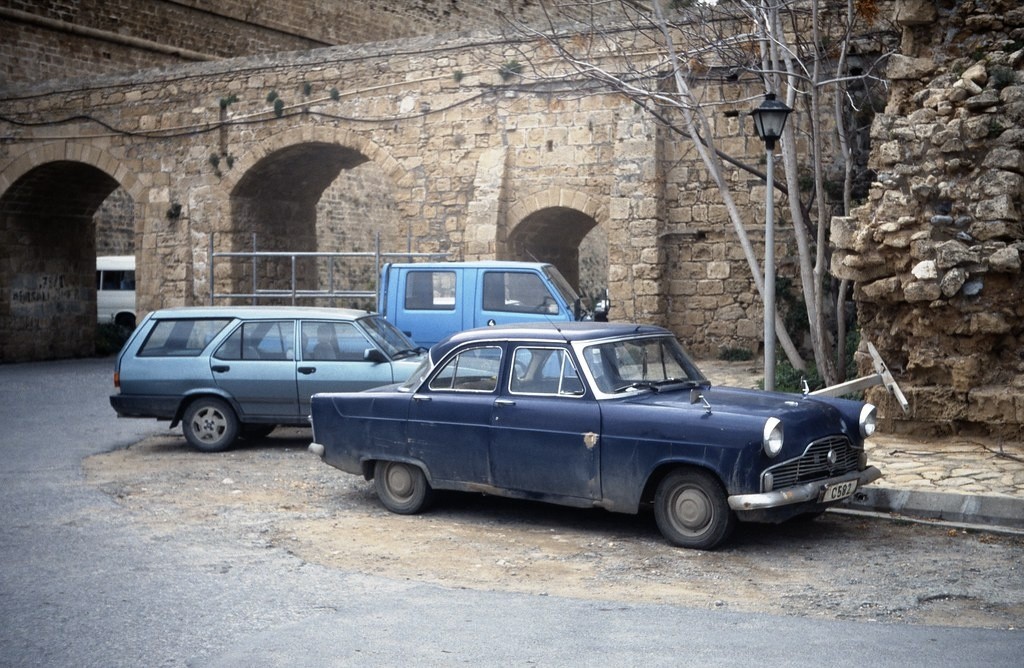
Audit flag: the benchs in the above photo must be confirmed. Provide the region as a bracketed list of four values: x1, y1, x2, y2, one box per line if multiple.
[204, 331, 260, 359]
[454, 379, 496, 391]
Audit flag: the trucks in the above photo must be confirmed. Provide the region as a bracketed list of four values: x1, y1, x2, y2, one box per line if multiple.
[210, 220, 641, 383]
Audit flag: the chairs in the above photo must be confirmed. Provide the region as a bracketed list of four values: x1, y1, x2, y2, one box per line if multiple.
[313, 325, 336, 359]
[286, 332, 311, 359]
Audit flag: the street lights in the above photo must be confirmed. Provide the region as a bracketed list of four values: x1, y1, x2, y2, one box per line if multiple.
[748, 92, 795, 390]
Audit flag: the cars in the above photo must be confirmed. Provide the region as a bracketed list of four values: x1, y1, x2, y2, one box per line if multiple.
[309, 322, 882, 550]
[110, 307, 525, 452]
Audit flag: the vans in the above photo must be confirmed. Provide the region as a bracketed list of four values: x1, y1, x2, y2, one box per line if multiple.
[96, 256, 136, 333]
